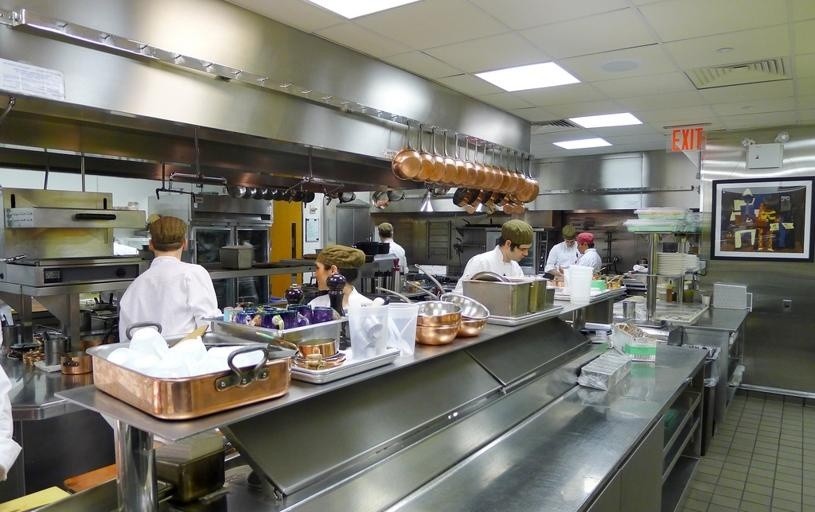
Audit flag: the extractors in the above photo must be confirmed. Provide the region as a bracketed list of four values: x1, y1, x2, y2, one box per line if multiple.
[367, 150, 705, 213]
[0, 1, 533, 193]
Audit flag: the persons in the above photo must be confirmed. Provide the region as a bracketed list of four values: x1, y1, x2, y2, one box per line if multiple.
[575, 233, 603, 272]
[118, 215, 223, 341]
[453, 219, 534, 300]
[308, 244, 370, 307]
[374, 223, 407, 271]
[544, 224, 581, 280]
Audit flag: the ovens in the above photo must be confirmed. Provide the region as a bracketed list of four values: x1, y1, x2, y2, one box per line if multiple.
[483, 227, 554, 275]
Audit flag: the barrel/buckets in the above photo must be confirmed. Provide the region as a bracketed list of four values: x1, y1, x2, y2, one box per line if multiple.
[43, 336, 72, 366]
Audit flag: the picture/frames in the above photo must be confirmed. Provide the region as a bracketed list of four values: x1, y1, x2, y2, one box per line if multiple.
[709, 176, 815, 261]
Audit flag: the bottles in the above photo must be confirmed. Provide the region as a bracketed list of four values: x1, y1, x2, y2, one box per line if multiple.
[284, 283, 302, 306]
[667, 283, 673, 302]
[326, 266, 348, 349]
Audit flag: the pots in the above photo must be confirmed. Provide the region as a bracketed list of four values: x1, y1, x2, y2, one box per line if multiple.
[392, 121, 540, 215]
[255, 331, 345, 370]
[60, 350, 92, 375]
[225, 186, 356, 208]
[376, 264, 489, 344]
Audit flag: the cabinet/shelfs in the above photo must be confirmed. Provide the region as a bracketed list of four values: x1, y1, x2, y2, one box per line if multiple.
[191, 227, 268, 311]
[661, 361, 706, 512]
[579, 415, 663, 512]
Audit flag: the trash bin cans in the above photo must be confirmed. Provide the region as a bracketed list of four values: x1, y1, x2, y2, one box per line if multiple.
[681, 378, 717, 456]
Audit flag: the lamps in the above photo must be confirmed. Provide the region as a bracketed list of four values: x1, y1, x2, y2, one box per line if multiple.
[740, 129, 792, 170]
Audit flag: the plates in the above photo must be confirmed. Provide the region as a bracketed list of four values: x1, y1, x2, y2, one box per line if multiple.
[657, 253, 700, 276]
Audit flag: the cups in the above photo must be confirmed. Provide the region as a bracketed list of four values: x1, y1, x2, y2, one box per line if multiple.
[348, 302, 418, 358]
[702, 295, 711, 306]
[564, 264, 595, 306]
[223, 301, 332, 330]
[3, 325, 17, 346]
[623, 300, 637, 318]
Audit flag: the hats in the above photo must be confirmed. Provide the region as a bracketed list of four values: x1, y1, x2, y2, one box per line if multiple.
[148, 215, 188, 246]
[576, 232, 595, 245]
[561, 224, 577, 240]
[500, 217, 534, 246]
[315, 243, 367, 271]
[377, 221, 394, 234]
